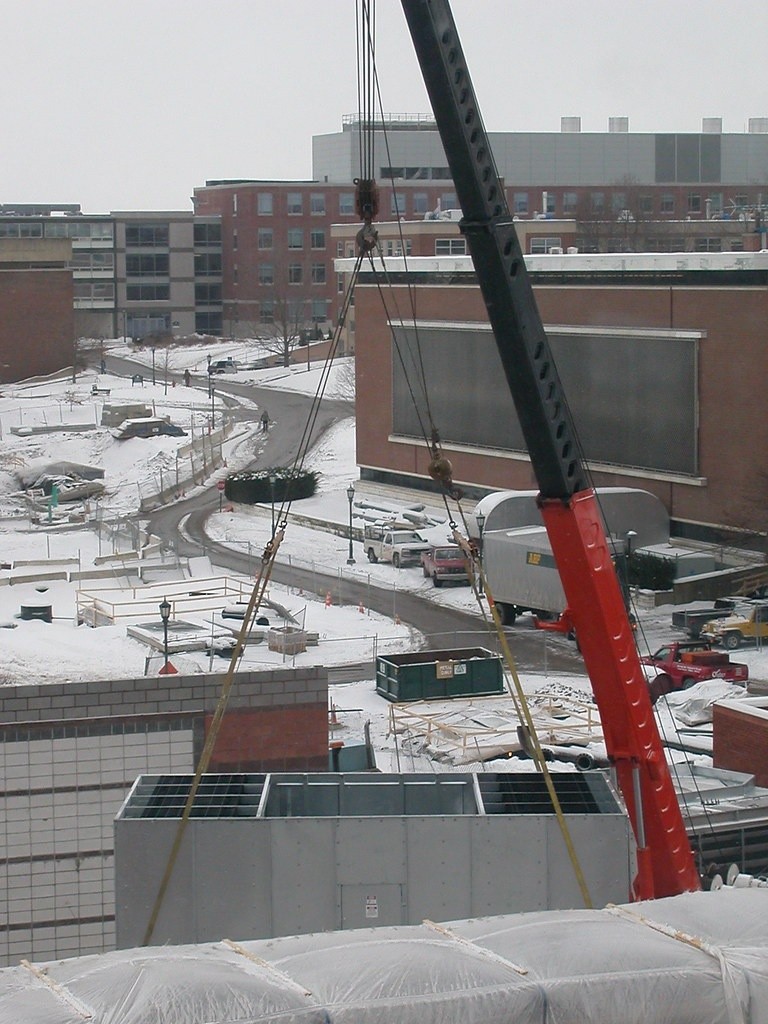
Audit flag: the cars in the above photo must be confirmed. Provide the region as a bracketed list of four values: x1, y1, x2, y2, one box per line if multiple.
[207, 360, 237, 375]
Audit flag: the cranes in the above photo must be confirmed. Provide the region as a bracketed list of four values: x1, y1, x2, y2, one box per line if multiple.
[358, 0, 701, 907]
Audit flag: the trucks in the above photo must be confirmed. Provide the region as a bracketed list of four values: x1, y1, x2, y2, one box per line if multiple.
[482, 523, 637, 643]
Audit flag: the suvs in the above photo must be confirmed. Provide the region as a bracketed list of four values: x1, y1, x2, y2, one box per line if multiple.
[700, 600, 768, 651]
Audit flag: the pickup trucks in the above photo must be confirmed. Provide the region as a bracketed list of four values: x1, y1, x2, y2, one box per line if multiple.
[420, 545, 477, 588]
[671, 595, 750, 639]
[641, 638, 750, 692]
[364, 530, 436, 568]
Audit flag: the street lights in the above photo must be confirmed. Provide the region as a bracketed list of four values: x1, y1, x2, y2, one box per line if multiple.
[476, 509, 487, 599]
[206, 353, 212, 399]
[151, 343, 155, 385]
[211, 381, 216, 430]
[159, 594, 171, 676]
[346, 484, 356, 566]
[626, 530, 639, 582]
